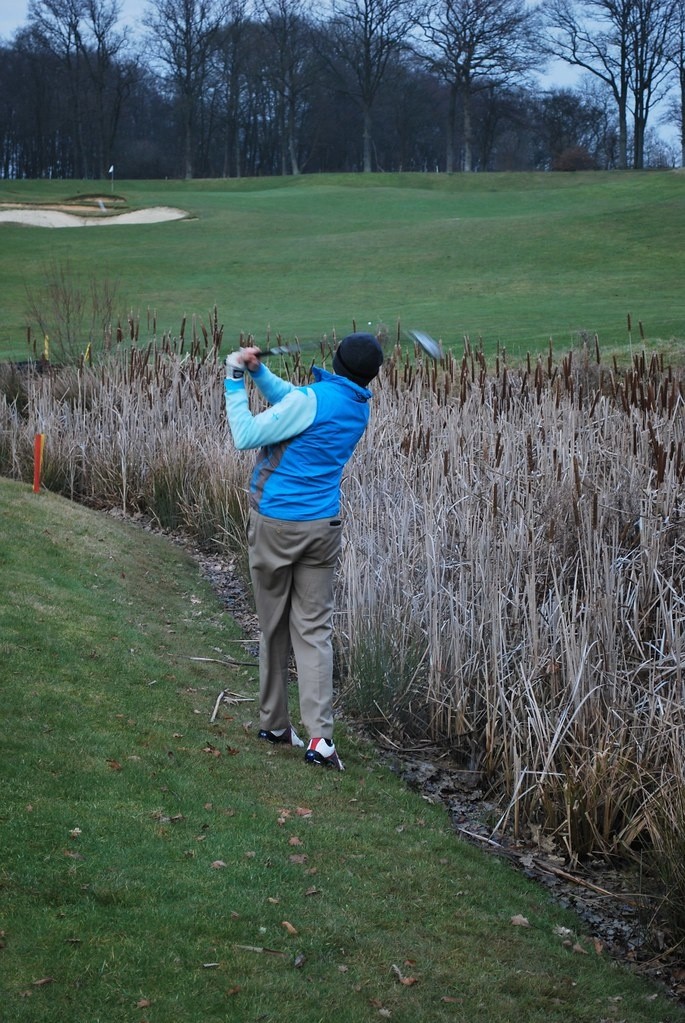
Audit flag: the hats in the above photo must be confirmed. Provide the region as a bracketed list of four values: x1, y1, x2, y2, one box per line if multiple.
[333, 331, 383, 386]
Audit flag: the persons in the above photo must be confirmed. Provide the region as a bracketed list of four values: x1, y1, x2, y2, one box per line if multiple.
[225, 334, 384, 772]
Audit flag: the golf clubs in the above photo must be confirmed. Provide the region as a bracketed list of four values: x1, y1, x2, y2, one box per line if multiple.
[223, 329, 442, 366]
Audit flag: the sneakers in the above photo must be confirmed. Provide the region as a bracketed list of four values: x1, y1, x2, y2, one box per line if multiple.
[304, 737, 346, 771]
[258, 724, 304, 748]
